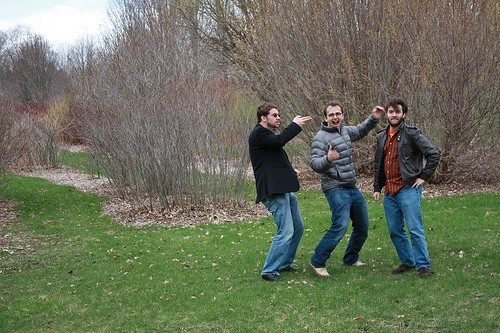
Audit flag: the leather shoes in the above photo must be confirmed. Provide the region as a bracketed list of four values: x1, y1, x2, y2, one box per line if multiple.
[352, 257, 368, 266]
[283, 265, 296, 271]
[391, 263, 415, 274]
[418, 267, 431, 278]
[308, 262, 331, 277]
[261, 274, 280, 281]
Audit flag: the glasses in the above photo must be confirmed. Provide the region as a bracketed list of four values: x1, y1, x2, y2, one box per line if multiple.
[326, 113, 344, 118]
[271, 113, 281, 117]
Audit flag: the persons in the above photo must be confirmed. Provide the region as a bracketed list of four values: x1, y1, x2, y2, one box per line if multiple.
[373, 98, 440, 280]
[310, 101, 385, 279]
[248, 102, 313, 281]
